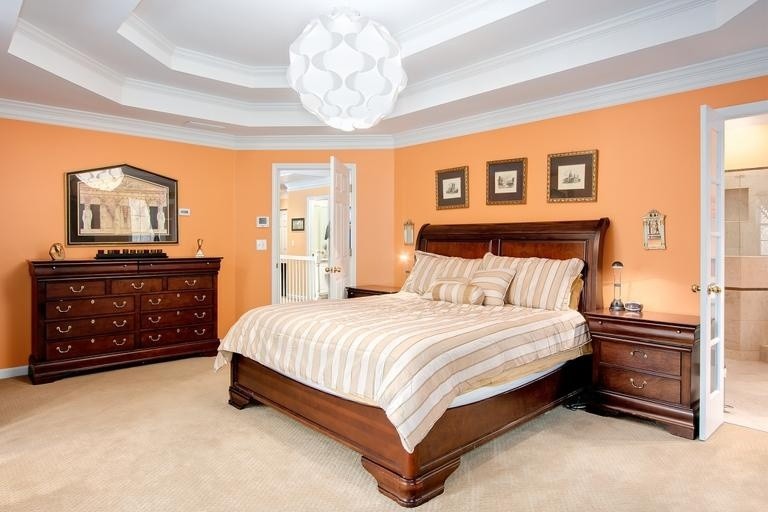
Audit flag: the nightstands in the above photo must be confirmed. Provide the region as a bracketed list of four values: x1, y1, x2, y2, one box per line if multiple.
[345, 284, 402, 298]
[582, 306, 716, 440]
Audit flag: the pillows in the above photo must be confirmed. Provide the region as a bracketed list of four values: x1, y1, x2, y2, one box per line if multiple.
[432, 275, 485, 305]
[470, 268, 518, 307]
[401, 249, 484, 296]
[478, 251, 585, 313]
[422, 276, 470, 303]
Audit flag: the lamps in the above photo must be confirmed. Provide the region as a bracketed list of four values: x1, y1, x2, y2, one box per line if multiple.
[286, 6, 409, 135]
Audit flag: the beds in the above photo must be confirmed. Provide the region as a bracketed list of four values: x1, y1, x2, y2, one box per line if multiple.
[213, 217, 610, 507]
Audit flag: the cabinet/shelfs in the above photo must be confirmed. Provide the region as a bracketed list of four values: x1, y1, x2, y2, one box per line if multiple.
[26, 256, 223, 386]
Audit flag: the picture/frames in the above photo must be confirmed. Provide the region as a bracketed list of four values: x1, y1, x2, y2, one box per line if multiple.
[435, 166, 470, 209]
[291, 218, 305, 231]
[546, 149, 599, 203]
[63, 163, 180, 246]
[485, 158, 528, 205]
